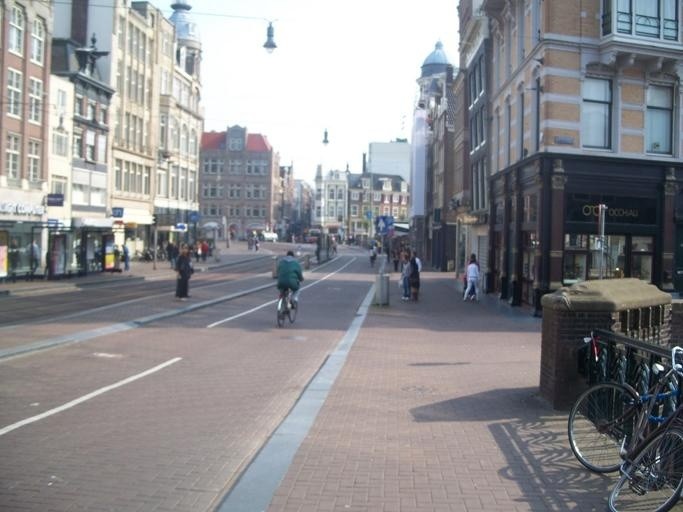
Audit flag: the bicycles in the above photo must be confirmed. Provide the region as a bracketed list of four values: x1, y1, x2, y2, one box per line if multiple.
[274, 279, 299, 329]
[565, 344, 683, 511]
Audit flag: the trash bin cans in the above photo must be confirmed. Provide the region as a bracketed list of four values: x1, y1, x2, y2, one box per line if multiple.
[377, 255, 388, 274]
[212, 248, 221, 263]
[375, 275, 389, 307]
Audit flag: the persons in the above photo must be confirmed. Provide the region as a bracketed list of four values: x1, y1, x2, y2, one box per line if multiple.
[463, 253, 480, 301]
[254, 236, 259, 253]
[120, 243, 130, 273]
[276, 251, 304, 320]
[366, 234, 422, 302]
[155, 236, 216, 272]
[174, 243, 194, 302]
[10, 238, 22, 279]
[460, 257, 481, 302]
[26, 237, 40, 277]
[110, 243, 120, 274]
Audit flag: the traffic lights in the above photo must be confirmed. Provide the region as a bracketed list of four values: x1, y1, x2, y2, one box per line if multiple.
[151, 213, 158, 226]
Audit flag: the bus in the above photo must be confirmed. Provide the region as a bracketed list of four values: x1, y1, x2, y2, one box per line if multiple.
[306, 228, 322, 242]
